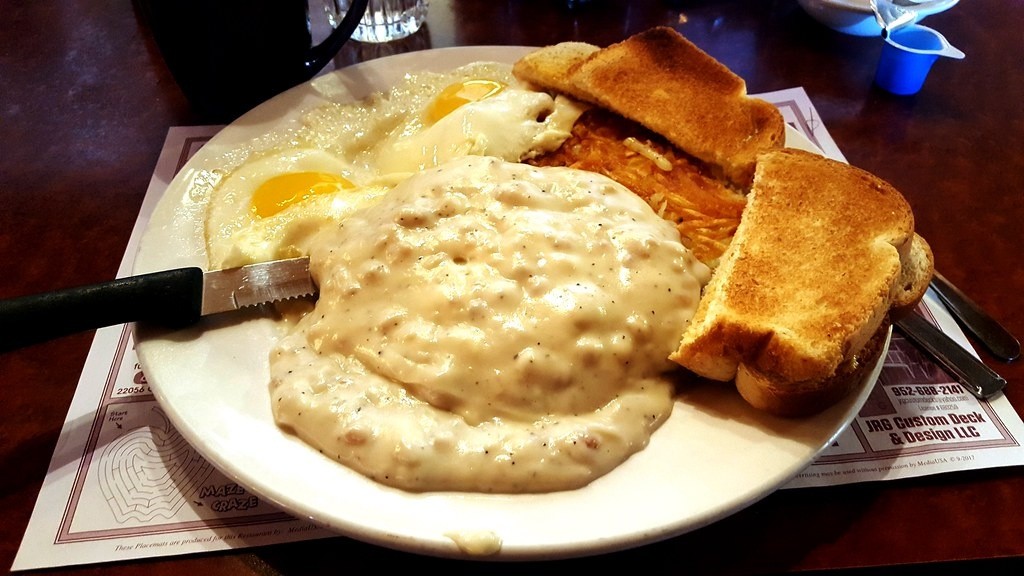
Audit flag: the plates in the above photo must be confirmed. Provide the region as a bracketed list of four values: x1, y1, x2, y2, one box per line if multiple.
[133, 48, 893, 556]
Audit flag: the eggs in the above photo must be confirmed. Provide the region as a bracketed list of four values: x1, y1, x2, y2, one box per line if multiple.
[177, 126, 398, 273]
[346, 58, 590, 185]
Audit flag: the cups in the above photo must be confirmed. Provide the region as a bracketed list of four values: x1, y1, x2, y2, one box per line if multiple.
[133, 0, 367, 124]
[323, 0, 429, 44]
[875, 24, 965, 96]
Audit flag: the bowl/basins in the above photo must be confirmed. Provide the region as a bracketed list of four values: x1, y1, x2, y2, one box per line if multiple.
[804, 0, 959, 36]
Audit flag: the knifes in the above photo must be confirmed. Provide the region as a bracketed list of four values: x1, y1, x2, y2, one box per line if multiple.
[0, 257, 320, 350]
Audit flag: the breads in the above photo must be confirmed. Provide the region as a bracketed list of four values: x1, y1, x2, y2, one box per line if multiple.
[509, 26, 786, 196]
[667, 146, 934, 419]
